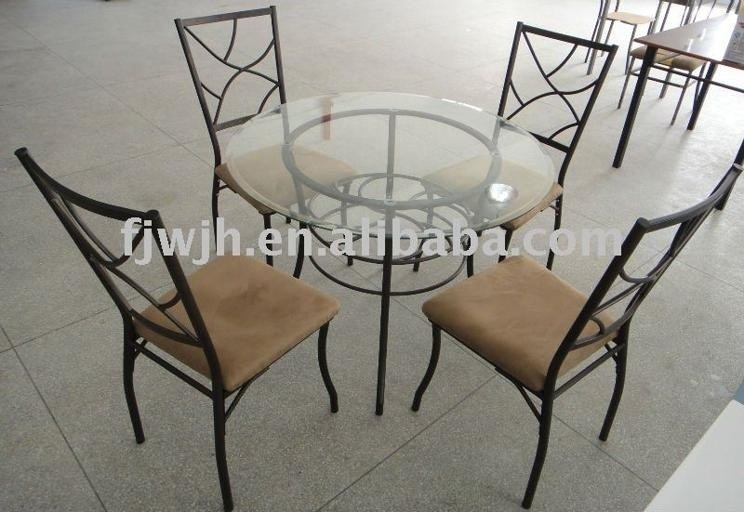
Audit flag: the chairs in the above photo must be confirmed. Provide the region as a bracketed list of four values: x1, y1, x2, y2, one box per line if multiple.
[412, 19, 617, 285]
[170, 3, 352, 277]
[399, 159, 742, 512]
[4, 143, 344, 510]
[579, 0, 739, 128]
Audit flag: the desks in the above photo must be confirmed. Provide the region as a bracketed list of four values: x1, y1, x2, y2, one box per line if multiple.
[612, 9, 743, 212]
[228, 89, 558, 416]
